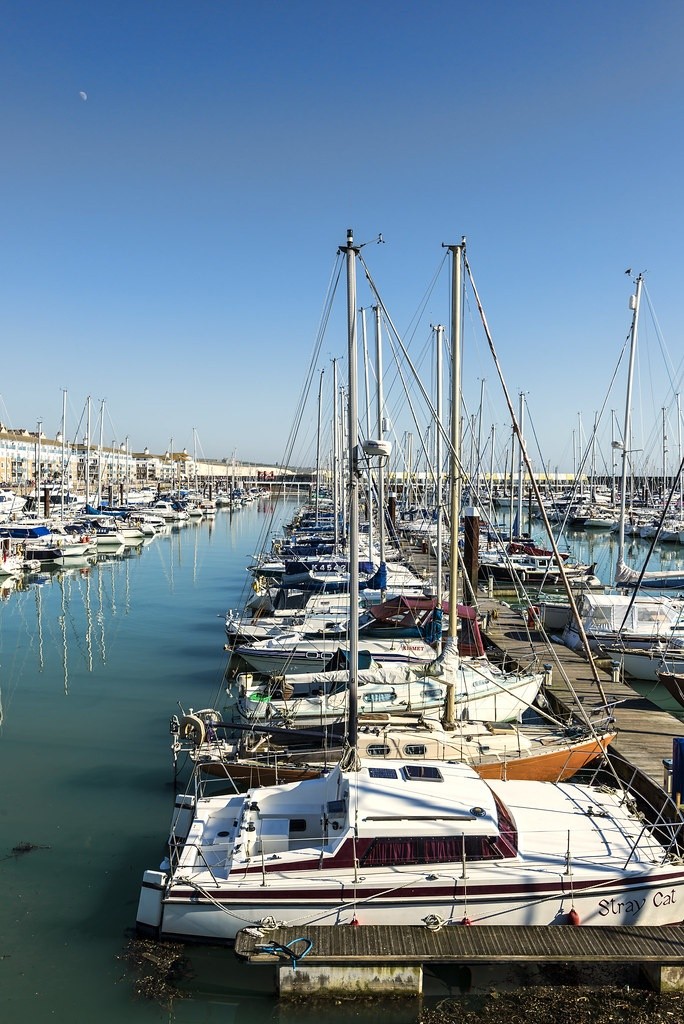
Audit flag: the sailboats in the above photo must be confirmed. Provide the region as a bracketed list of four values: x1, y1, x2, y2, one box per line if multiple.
[0, 536, 155, 725]
[0, 386, 274, 593]
[135, 226, 684, 945]
